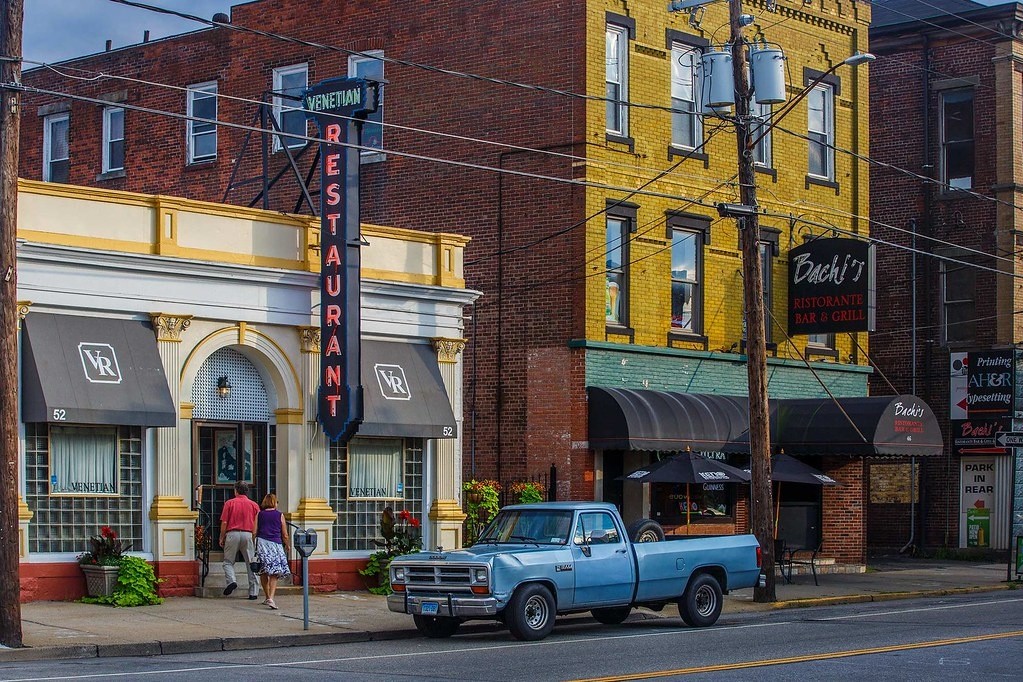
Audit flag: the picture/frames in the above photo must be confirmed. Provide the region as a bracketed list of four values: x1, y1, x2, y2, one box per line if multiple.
[212, 427, 257, 487]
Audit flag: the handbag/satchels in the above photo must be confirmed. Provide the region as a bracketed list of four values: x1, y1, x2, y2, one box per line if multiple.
[249, 552, 262, 573]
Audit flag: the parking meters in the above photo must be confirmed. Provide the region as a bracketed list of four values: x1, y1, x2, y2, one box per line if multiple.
[294, 527, 317, 630]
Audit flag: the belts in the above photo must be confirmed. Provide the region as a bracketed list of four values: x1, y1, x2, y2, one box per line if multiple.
[226, 529, 252, 533]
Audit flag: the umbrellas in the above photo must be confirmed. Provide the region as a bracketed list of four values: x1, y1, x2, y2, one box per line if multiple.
[612, 445, 852, 539]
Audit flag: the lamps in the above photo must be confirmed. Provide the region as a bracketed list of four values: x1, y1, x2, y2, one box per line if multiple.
[217, 375, 231, 398]
[730, 342, 737, 353]
[849, 353, 854, 364]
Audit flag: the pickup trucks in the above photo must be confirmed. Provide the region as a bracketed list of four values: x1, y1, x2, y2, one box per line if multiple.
[385, 498, 768, 642]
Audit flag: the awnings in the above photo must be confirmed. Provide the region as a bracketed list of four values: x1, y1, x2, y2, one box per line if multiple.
[21, 312, 176, 427]
[587, 384, 945, 458]
[355, 339, 458, 438]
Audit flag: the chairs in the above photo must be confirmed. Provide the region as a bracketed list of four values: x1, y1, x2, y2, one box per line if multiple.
[774, 538, 787, 584]
[779, 538, 826, 586]
[590, 530, 610, 543]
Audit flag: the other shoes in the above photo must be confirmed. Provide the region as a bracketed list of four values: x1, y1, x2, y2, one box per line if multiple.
[223, 582, 238, 595]
[249, 595, 257, 600]
[262, 599, 268, 605]
[268, 599, 278, 609]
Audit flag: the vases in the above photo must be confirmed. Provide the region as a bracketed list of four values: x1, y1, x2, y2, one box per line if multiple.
[467, 494, 483, 504]
[80, 564, 119, 598]
[378, 558, 390, 586]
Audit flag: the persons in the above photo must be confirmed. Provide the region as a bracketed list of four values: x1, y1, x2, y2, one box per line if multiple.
[252, 494, 291, 609]
[220, 481, 260, 600]
[217, 434, 250, 482]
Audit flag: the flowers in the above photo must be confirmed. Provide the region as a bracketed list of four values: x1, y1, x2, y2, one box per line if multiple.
[462, 479, 503, 537]
[357, 506, 425, 596]
[508, 481, 546, 504]
[74, 527, 169, 607]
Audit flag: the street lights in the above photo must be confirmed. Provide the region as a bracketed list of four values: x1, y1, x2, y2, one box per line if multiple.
[737, 52, 878, 599]
